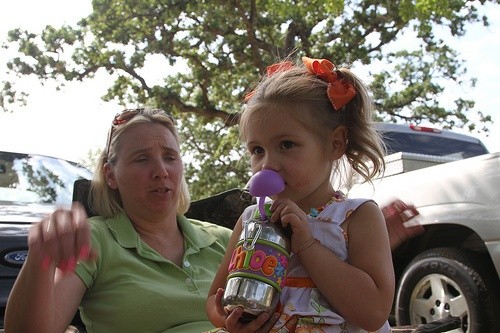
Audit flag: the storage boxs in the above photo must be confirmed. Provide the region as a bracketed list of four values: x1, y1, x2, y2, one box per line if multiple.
[358, 152, 456, 182]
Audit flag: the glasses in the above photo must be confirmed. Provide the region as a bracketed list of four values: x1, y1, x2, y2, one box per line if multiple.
[105, 107, 175, 164]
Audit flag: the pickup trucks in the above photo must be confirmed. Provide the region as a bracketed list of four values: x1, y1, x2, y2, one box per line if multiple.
[330, 121, 500, 333]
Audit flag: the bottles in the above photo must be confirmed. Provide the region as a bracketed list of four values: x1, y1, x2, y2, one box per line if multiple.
[221, 169, 292, 323]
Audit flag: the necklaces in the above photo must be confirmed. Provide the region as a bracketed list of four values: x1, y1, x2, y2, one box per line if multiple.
[308, 190, 344, 217]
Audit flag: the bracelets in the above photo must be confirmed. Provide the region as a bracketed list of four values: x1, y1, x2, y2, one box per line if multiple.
[294, 239, 318, 254]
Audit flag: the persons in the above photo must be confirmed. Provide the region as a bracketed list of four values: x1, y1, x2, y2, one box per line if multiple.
[3, 107, 424, 333]
[206, 54, 396, 333]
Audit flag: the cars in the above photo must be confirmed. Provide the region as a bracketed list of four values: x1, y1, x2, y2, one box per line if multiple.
[1, 152, 95, 310]
